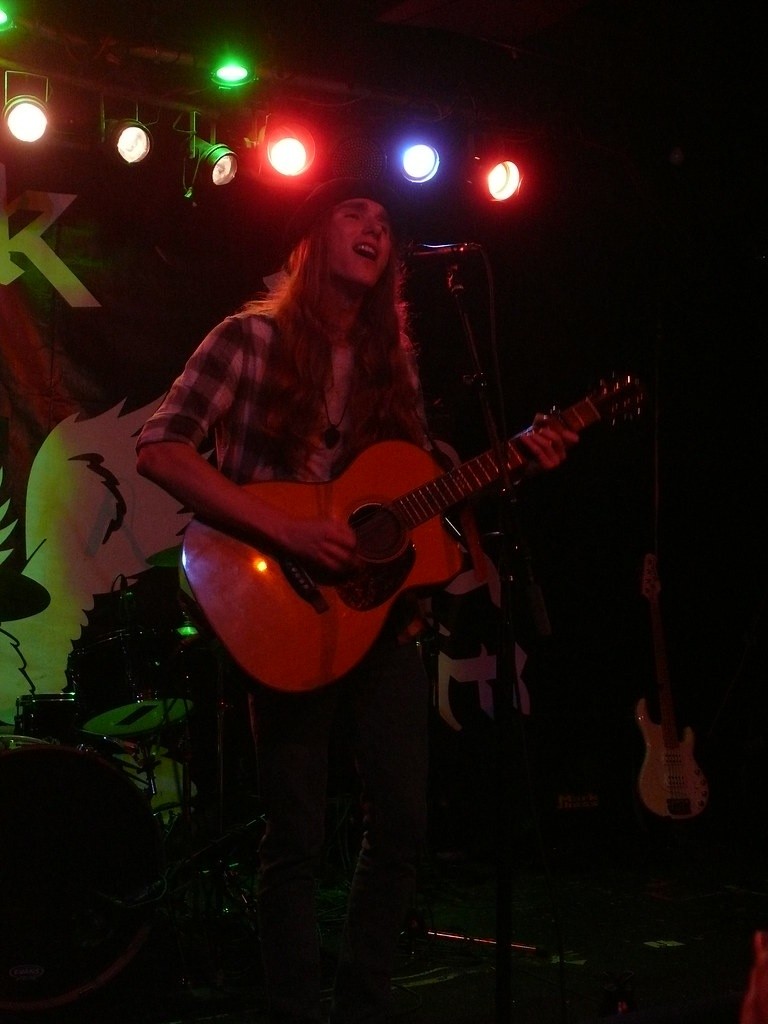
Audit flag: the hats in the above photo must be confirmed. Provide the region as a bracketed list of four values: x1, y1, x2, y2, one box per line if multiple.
[283, 177, 388, 245]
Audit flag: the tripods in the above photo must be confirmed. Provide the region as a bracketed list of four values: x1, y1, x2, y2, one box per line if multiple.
[177, 650, 256, 991]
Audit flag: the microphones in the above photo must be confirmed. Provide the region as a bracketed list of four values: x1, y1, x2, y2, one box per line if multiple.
[118, 576, 139, 625]
[404, 243, 481, 273]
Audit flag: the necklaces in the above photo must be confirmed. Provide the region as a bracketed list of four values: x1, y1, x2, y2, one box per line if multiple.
[320, 340, 358, 449]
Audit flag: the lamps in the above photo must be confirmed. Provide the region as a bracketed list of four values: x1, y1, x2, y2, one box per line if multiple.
[171, 111, 241, 200]
[0, 69, 59, 153]
[95, 92, 161, 166]
[244, 109, 318, 190]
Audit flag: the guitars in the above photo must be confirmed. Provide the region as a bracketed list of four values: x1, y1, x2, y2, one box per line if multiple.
[635, 536, 714, 824]
[177, 366, 651, 697]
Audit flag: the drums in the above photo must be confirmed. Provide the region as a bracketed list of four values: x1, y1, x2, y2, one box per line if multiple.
[65, 621, 197, 737]
[10, 690, 83, 744]
[0, 731, 171, 1015]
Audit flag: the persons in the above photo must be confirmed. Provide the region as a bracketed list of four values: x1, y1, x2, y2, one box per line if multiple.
[135, 174, 583, 1024]
